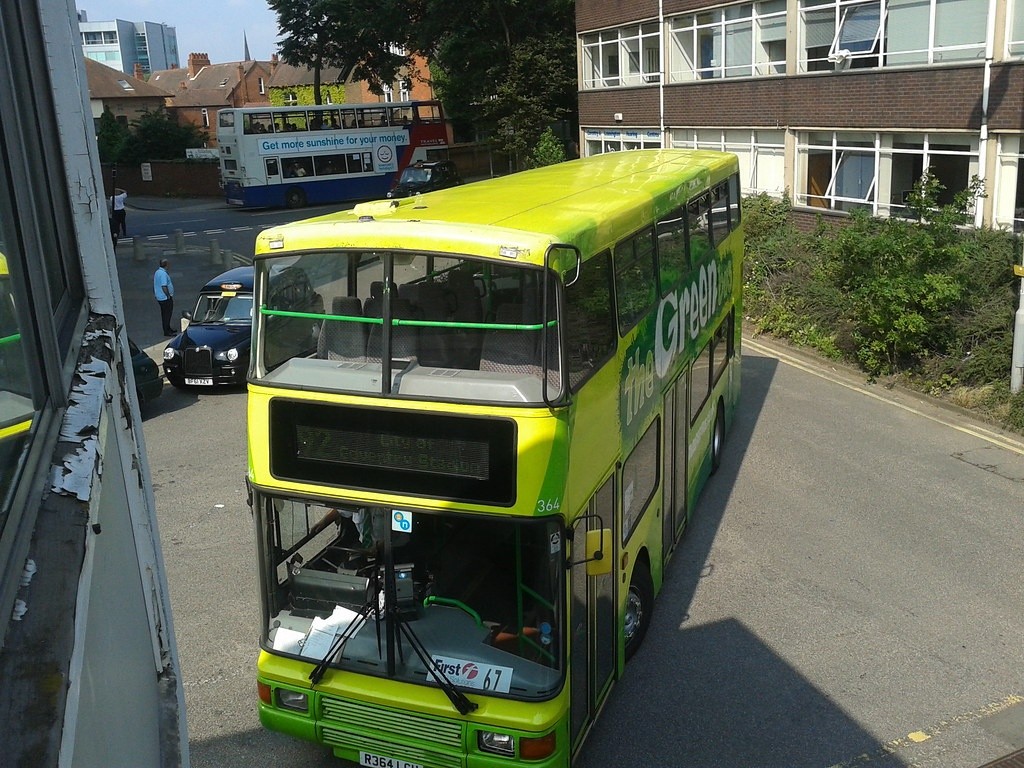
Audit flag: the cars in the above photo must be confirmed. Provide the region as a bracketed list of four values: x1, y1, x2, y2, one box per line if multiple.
[127, 336, 164, 405]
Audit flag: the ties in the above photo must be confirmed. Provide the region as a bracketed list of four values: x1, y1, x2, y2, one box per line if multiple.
[362, 507, 372, 547]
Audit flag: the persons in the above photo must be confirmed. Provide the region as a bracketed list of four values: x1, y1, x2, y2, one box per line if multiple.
[409, 174, 421, 181]
[351, 119, 363, 127]
[401, 116, 409, 124]
[109, 188, 128, 236]
[285, 123, 297, 131]
[153, 258, 177, 336]
[380, 116, 387, 126]
[294, 163, 306, 176]
[251, 121, 280, 133]
[309, 117, 347, 129]
[493, 594, 555, 667]
[308, 508, 386, 596]
[569, 139, 579, 158]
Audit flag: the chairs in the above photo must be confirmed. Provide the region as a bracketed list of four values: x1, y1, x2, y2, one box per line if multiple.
[313, 258, 559, 386]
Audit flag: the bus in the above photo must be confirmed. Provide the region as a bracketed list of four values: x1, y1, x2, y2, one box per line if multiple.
[244, 149, 747, 768]
[0, 250, 37, 448]
[214, 99, 452, 211]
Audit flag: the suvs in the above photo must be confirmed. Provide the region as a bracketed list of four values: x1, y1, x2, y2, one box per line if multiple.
[167, 262, 327, 388]
[386, 160, 465, 200]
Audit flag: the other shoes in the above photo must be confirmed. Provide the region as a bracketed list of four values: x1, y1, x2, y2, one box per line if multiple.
[170, 329, 177, 333]
[164, 333, 177, 336]
